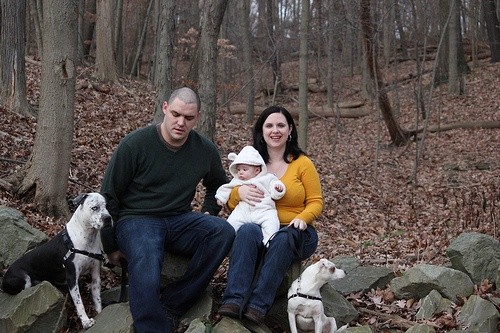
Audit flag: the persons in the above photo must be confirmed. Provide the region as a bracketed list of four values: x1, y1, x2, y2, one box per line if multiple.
[218, 106, 323, 324]
[99, 88, 236, 333]
[215, 146, 286, 249]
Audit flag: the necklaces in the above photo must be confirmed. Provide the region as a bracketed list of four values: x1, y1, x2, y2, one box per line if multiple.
[273, 170, 276, 175]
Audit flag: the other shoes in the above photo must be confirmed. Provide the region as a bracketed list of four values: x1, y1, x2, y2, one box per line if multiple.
[162, 306, 180, 330]
[218, 301, 242, 318]
[244, 306, 266, 326]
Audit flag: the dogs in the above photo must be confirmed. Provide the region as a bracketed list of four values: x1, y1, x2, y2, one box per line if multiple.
[287, 259, 350, 333]
[1, 192, 113, 329]
